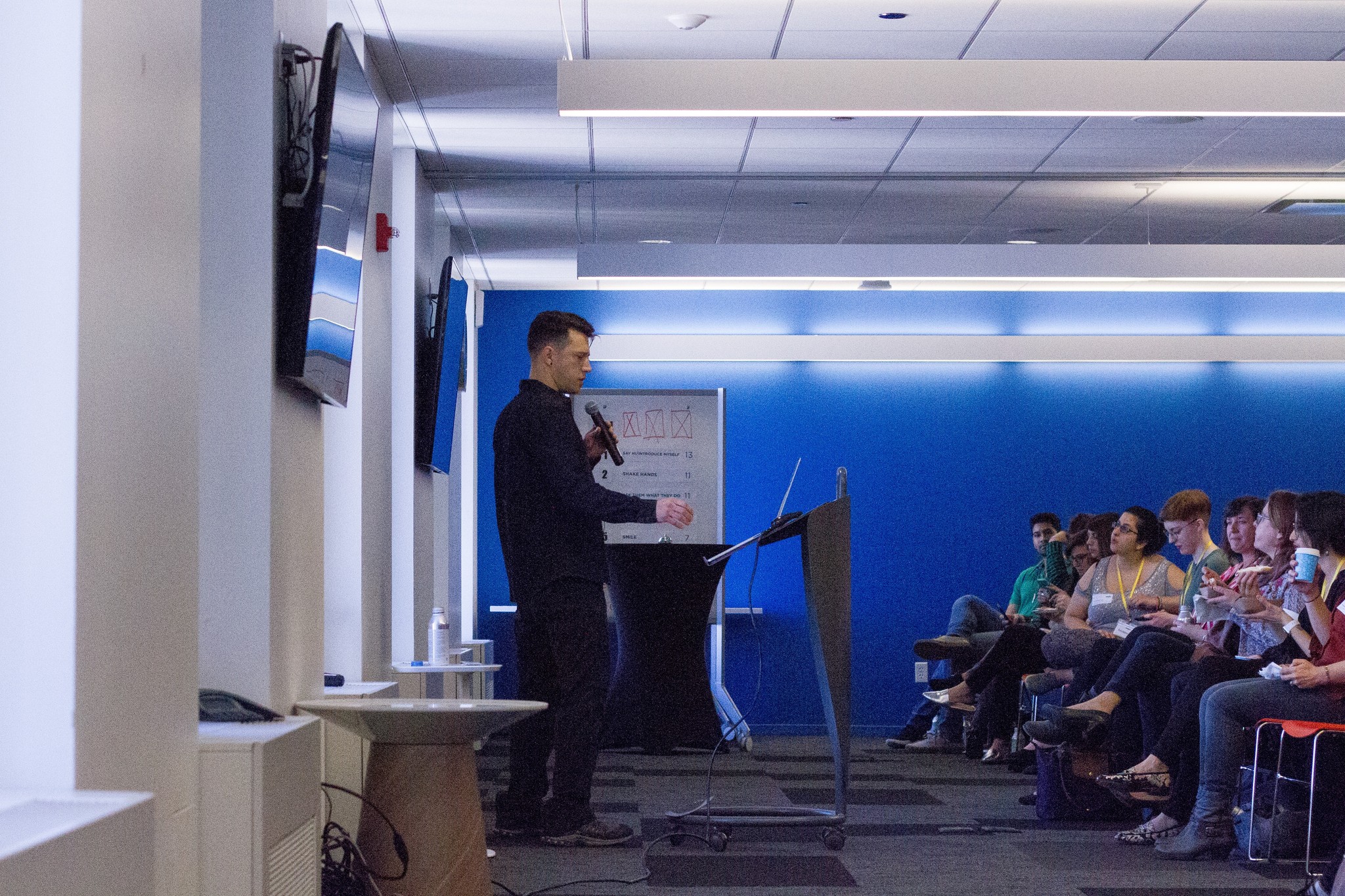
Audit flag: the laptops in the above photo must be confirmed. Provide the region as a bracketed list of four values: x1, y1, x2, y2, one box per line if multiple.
[708, 458, 801, 560]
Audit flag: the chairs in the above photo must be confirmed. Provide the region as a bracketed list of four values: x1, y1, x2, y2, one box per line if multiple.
[1015, 673, 1071, 754]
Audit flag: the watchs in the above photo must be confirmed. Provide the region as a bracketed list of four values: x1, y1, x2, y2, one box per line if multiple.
[1282, 619, 1301, 634]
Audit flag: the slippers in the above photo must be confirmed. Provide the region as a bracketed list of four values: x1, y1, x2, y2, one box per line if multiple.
[981, 748, 1010, 763]
[922, 688, 976, 713]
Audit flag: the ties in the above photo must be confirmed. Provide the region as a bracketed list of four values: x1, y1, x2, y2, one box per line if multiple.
[1110, 521, 1140, 534]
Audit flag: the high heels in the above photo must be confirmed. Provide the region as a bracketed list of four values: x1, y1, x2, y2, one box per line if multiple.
[1154, 818, 1235, 861]
[1041, 704, 1110, 740]
[1022, 720, 1098, 752]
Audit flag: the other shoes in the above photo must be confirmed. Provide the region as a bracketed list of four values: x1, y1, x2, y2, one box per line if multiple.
[1296, 873, 1328, 896]
[913, 634, 971, 661]
[1024, 672, 1058, 695]
[1109, 789, 1173, 815]
[885, 725, 927, 748]
[1008, 750, 1036, 772]
[905, 736, 966, 754]
[1095, 766, 1171, 796]
[542, 808, 634, 846]
[1115, 821, 1189, 845]
[965, 730, 982, 759]
[492, 791, 546, 836]
[1018, 792, 1036, 806]
[1085, 797, 1129, 820]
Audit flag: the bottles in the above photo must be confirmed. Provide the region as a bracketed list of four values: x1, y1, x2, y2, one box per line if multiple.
[1177, 605, 1193, 627]
[428, 607, 451, 666]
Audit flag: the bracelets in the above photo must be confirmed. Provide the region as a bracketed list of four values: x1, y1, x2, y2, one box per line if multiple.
[1156, 595, 1162, 611]
[1304, 593, 1321, 603]
[1323, 666, 1331, 686]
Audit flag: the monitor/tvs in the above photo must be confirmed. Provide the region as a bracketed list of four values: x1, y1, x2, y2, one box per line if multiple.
[277, 23, 379, 409]
[418, 255, 469, 477]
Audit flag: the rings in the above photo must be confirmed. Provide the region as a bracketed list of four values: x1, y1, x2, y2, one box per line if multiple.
[1041, 593, 1045, 598]
[1290, 681, 1293, 685]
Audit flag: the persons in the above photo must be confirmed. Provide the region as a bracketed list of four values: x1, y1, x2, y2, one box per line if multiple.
[487, 312, 696, 849]
[884, 489, 1345, 860]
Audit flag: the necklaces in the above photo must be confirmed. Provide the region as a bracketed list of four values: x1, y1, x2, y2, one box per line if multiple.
[1234, 555, 1260, 576]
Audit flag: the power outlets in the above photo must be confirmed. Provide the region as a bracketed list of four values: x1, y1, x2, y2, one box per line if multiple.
[278, 29, 294, 85]
[429, 277, 432, 305]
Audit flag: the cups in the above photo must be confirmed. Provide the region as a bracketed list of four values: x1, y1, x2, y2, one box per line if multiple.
[1294, 548, 1321, 583]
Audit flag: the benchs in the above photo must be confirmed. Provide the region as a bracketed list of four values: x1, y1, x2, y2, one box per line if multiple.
[1236, 718, 1345, 878]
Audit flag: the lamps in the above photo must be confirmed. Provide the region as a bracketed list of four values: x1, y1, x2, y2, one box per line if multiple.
[586, 334, 1345, 362]
[575, 184, 1345, 294]
[554, 0, 1345, 117]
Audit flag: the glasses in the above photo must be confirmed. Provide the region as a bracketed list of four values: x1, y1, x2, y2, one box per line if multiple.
[1069, 552, 1089, 565]
[1257, 512, 1270, 524]
[1163, 519, 1197, 539]
[1292, 520, 1304, 533]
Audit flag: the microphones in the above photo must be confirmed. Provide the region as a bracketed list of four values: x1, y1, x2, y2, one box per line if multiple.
[584, 400, 624, 466]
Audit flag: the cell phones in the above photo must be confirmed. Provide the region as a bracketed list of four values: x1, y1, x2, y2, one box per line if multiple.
[1037, 579, 1051, 589]
[1134, 616, 1150, 621]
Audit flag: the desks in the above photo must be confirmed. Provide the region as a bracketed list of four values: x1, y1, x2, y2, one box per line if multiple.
[490, 605, 762, 752]
[294, 697, 549, 896]
